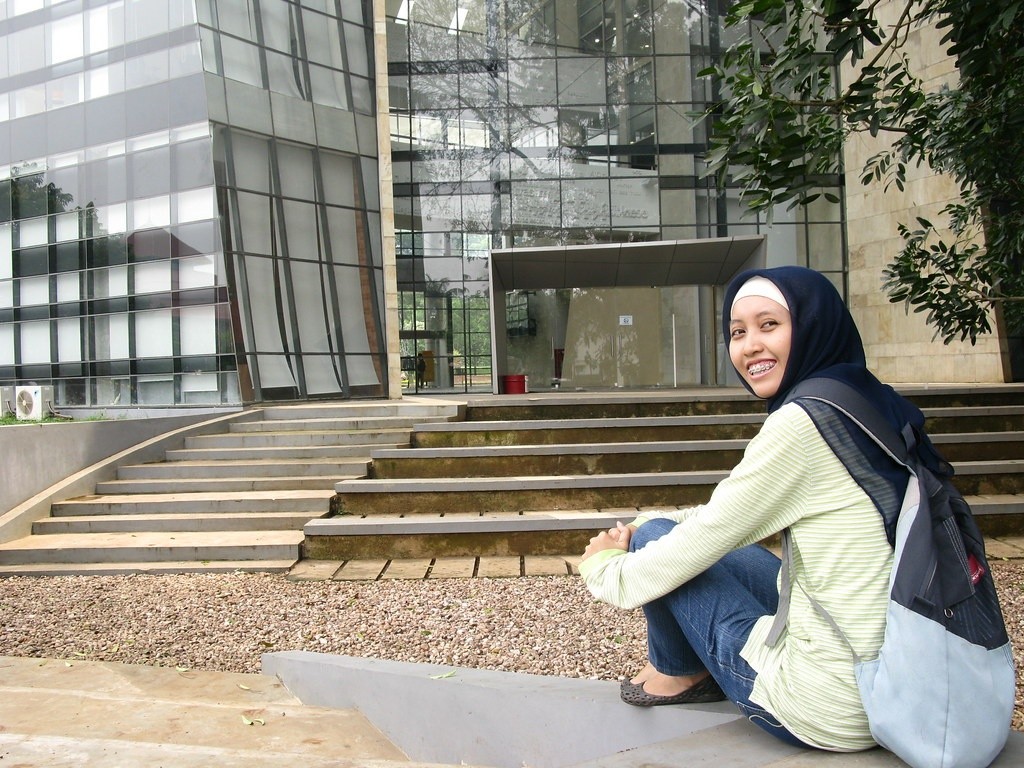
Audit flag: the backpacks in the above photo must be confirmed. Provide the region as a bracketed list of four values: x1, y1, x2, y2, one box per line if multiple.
[778, 377, 1016, 768]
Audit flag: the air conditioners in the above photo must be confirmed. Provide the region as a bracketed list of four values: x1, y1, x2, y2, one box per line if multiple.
[15, 385, 54, 422]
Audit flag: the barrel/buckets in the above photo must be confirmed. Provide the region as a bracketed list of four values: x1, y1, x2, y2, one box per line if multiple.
[503, 374, 530, 393]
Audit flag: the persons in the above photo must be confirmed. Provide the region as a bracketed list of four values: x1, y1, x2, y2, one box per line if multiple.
[417, 353, 426, 389]
[574, 266, 939, 752]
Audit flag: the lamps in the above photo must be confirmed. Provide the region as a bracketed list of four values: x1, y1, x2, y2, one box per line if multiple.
[594, 9, 650, 48]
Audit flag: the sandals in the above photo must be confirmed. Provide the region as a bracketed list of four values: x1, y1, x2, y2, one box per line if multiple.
[620, 674, 727, 706]
